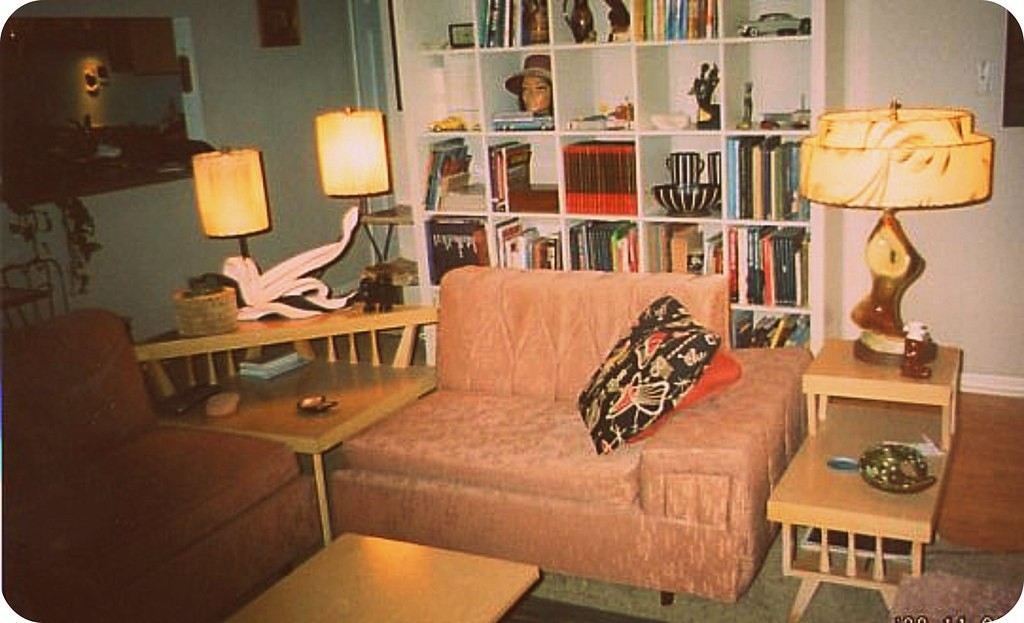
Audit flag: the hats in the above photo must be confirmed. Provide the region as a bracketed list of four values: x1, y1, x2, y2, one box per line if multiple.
[504, 55, 552, 95]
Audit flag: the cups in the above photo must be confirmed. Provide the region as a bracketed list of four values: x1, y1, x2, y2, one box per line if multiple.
[666, 152, 704, 185]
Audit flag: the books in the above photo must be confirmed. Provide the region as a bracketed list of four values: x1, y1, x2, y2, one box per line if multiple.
[236, 349, 299, 379]
[425, 0, 810, 350]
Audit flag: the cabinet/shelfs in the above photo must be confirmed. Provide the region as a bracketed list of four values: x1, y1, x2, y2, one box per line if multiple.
[392, 0, 844, 366]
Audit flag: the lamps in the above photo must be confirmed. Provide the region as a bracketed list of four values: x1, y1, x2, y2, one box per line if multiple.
[800, 93, 996, 366]
[192, 144, 333, 318]
[316, 107, 392, 314]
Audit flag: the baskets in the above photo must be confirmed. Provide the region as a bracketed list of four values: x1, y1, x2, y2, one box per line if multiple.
[174, 286, 239, 336]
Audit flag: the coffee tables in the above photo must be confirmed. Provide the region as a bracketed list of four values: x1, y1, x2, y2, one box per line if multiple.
[224, 532, 540, 623]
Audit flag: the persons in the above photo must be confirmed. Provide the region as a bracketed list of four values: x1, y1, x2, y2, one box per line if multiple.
[503, 54, 554, 118]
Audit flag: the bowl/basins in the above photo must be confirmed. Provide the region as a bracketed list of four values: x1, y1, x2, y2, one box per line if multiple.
[651, 183, 719, 214]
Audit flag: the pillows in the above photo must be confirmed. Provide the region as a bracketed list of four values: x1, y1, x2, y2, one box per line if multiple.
[626, 350, 742, 445]
[576, 295, 721, 456]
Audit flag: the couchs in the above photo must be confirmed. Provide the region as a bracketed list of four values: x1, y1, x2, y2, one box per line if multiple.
[330, 264, 813, 606]
[0, 307, 319, 623]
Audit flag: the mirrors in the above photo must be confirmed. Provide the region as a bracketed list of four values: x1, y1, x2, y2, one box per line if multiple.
[0, 17, 207, 206]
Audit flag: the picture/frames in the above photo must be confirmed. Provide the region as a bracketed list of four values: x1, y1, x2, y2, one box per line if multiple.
[254, 0, 304, 48]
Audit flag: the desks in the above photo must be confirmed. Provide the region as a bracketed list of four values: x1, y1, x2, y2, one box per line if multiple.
[136, 302, 437, 397]
[156, 359, 437, 547]
[767, 403, 952, 623]
[801, 338, 964, 452]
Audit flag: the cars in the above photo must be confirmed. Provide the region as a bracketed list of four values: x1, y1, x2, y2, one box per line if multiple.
[739, 12, 802, 37]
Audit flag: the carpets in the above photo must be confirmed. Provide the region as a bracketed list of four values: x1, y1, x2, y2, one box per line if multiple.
[935, 393, 1023, 551]
